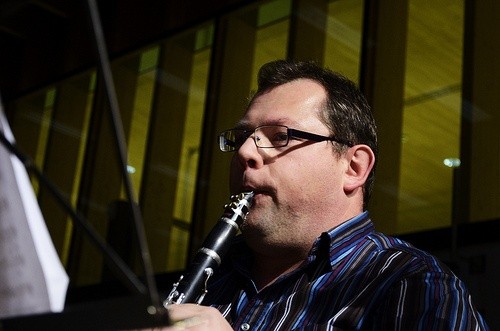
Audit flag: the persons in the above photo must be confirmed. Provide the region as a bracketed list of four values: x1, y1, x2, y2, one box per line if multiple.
[144, 59, 492, 331]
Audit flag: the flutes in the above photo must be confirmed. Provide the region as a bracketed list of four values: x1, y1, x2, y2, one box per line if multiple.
[161, 187, 256, 314]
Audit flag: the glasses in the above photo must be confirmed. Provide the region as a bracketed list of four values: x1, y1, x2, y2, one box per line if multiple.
[217, 125, 353, 152]
